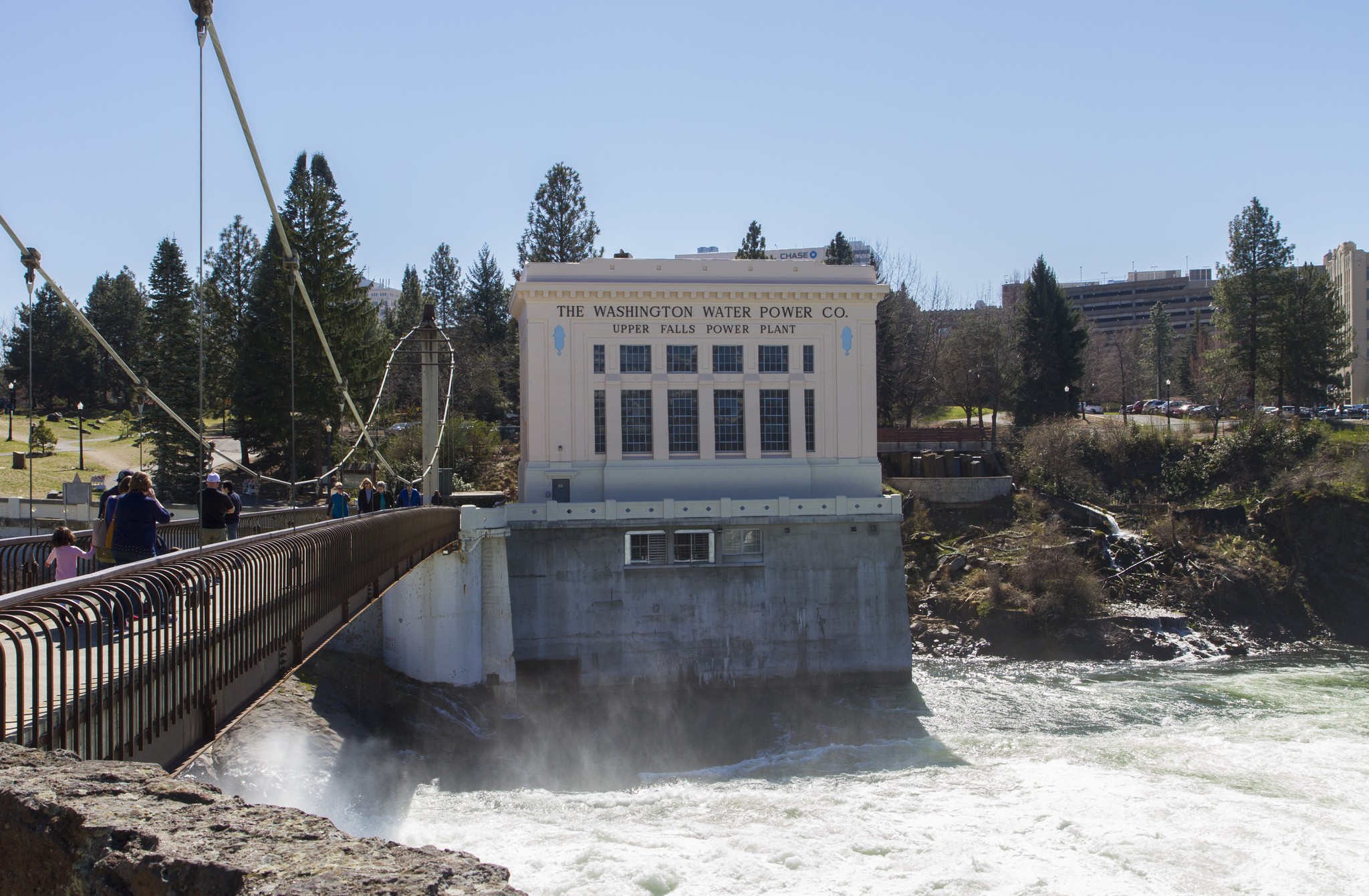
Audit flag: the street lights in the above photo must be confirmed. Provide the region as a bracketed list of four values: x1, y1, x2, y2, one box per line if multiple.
[1166, 379, 1170, 431]
[326, 424, 333, 504]
[1208, 303, 1223, 382]
[221, 388, 227, 436]
[6, 382, 14, 442]
[77, 402, 84, 471]
[1064, 385, 1069, 412]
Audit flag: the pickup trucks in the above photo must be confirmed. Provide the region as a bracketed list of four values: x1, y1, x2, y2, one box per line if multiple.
[1347, 404, 1369, 420]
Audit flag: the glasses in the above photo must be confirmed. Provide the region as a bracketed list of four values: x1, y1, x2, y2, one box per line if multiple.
[337, 488, 343, 490]
[221, 488, 226, 490]
[364, 482, 369, 485]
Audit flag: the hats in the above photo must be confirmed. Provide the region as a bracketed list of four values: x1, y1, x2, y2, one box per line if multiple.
[113, 469, 134, 482]
[207, 472, 221, 482]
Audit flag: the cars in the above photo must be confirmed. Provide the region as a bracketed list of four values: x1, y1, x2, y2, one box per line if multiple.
[438, 419, 448, 428]
[1078, 402, 1104, 415]
[385, 421, 423, 436]
[1119, 399, 1233, 421]
[1260, 404, 1355, 419]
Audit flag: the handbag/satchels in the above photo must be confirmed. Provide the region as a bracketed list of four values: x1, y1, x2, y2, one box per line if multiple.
[92, 507, 107, 547]
[105, 494, 124, 548]
[95, 547, 116, 563]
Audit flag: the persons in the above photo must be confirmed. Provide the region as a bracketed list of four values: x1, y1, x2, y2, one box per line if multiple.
[44, 526, 97, 627]
[196, 472, 235, 586]
[373, 481, 396, 512]
[431, 490, 442, 506]
[97, 469, 188, 632]
[396, 483, 422, 508]
[326, 482, 351, 520]
[358, 478, 377, 513]
[221, 480, 244, 569]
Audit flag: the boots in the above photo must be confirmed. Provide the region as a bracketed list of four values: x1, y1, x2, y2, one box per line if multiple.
[69, 607, 84, 624]
[58, 611, 71, 627]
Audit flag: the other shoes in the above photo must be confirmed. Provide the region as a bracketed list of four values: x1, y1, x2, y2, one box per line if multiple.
[113, 621, 129, 633]
[101, 616, 109, 620]
[160, 614, 178, 628]
[133, 609, 148, 616]
[216, 577, 220, 585]
[125, 615, 139, 622]
[205, 578, 213, 586]
[227, 564, 244, 570]
